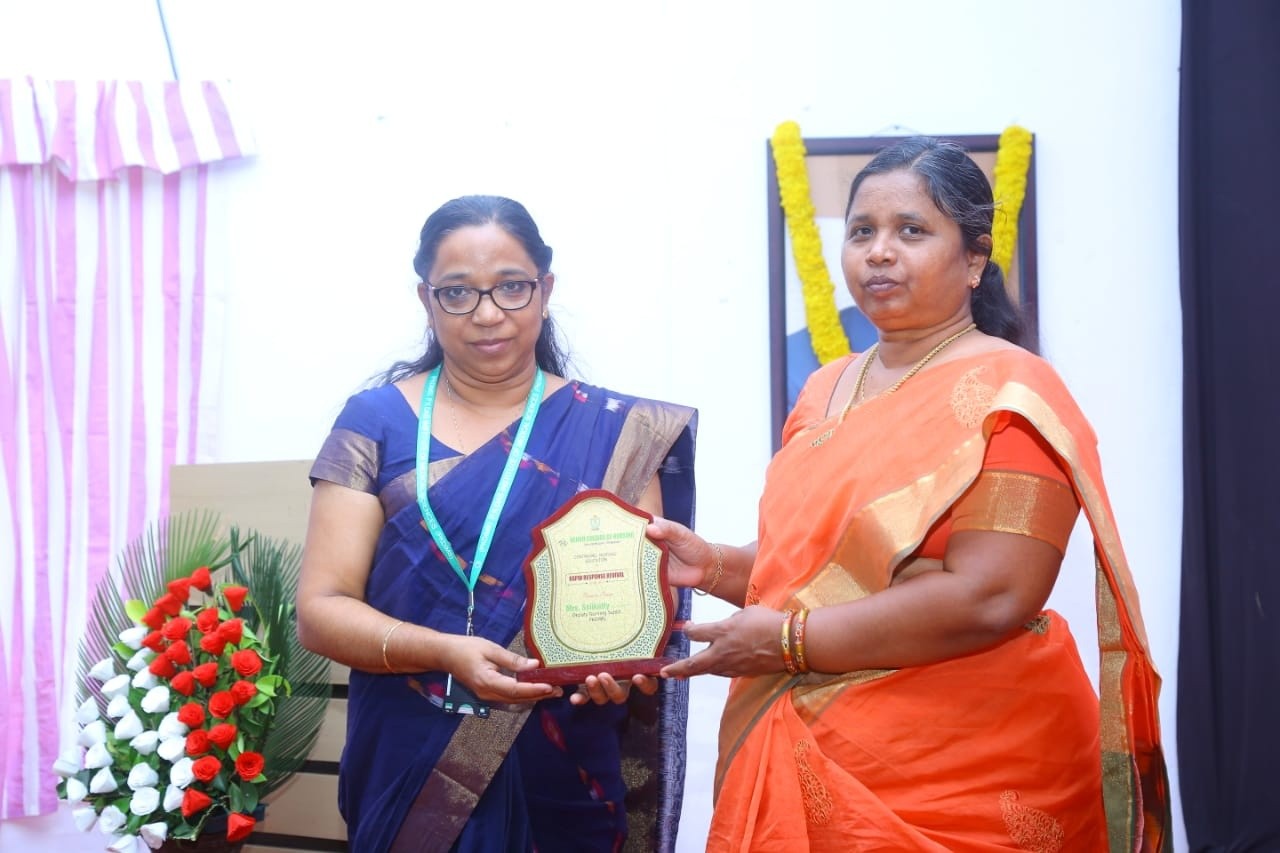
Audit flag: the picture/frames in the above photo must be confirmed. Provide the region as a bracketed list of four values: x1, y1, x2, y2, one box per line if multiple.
[767, 134, 1038, 458]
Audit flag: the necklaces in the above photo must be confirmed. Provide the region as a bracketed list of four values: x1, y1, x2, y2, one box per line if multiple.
[808, 322, 977, 448]
[442, 370, 531, 455]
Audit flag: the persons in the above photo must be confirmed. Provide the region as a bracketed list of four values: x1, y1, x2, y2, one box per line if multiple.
[643, 134, 1114, 853]
[293, 195, 682, 853]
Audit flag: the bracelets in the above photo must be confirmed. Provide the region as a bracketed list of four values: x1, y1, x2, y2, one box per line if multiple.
[780, 607, 811, 676]
[382, 620, 404, 674]
[692, 543, 724, 596]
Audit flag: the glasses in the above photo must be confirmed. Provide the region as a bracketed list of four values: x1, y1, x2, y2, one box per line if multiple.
[424, 276, 539, 315]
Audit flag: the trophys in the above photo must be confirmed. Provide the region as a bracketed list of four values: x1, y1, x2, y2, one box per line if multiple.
[508, 486, 678, 687]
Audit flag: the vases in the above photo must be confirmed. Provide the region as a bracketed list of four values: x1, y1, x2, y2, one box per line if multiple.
[151, 832, 245, 853]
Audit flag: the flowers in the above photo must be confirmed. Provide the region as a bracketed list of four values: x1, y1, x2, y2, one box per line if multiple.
[53, 508, 331, 853]
